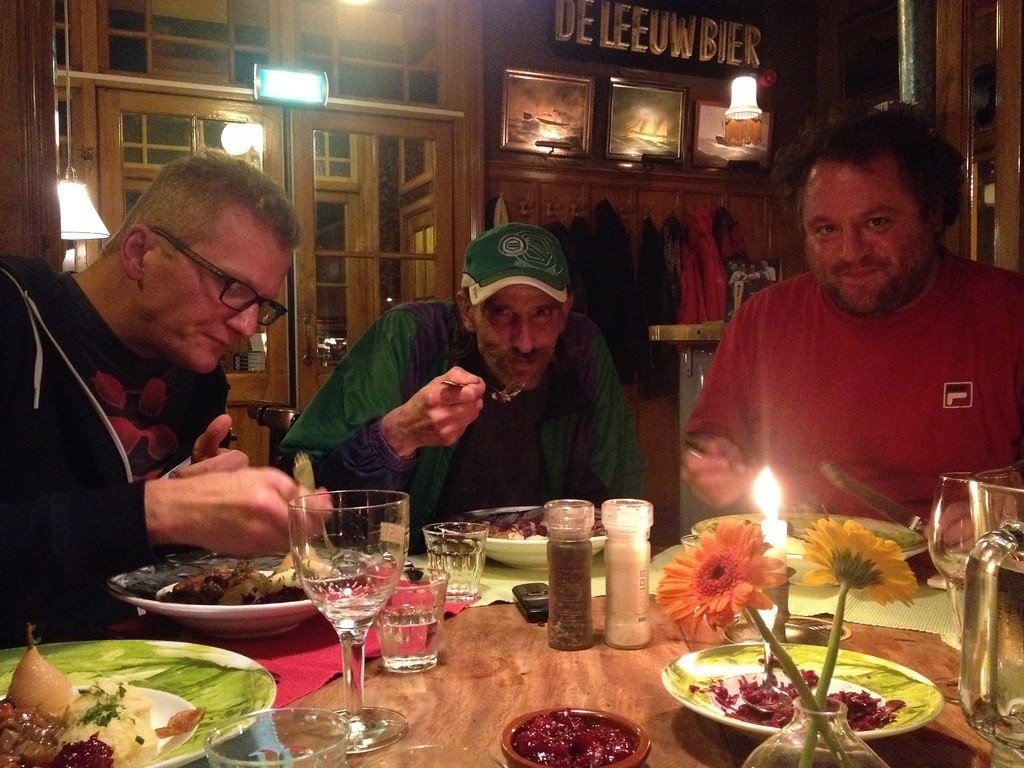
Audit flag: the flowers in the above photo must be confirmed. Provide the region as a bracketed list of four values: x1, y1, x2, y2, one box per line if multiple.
[655, 514, 921, 768]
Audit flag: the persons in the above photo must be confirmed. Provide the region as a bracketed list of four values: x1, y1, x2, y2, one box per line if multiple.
[678, 85, 1024, 568]
[0, 145, 334, 651]
[726, 258, 776, 319]
[276, 221, 645, 556]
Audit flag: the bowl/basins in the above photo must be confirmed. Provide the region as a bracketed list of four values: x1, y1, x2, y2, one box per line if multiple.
[443, 504, 617, 570]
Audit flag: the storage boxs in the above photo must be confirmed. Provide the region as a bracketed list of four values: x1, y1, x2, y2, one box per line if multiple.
[232, 332, 267, 372]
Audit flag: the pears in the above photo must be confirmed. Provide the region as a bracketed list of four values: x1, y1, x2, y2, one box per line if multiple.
[7, 622, 72, 716]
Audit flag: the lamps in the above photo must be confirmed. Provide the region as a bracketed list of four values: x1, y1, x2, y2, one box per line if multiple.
[726, 160, 761, 176]
[252, 62, 329, 109]
[724, 75, 763, 122]
[535, 140, 572, 159]
[640, 154, 677, 174]
[56, 163, 111, 239]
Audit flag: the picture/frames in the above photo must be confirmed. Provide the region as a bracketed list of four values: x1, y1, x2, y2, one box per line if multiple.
[691, 99, 776, 170]
[604, 77, 688, 163]
[498, 66, 595, 160]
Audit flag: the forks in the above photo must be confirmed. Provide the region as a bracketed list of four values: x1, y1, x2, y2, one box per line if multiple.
[439, 377, 527, 399]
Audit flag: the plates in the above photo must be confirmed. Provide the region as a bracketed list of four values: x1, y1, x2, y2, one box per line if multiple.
[0, 638, 277, 768]
[499, 706, 651, 768]
[689, 511, 930, 587]
[104, 548, 388, 637]
[661, 643, 944, 746]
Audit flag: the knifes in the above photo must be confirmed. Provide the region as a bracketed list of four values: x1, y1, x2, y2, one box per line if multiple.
[819, 462, 932, 541]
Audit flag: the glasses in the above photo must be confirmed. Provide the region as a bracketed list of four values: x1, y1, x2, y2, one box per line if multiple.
[142, 223, 289, 329]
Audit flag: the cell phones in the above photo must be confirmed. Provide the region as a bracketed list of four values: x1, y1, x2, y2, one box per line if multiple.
[510, 582, 551, 627]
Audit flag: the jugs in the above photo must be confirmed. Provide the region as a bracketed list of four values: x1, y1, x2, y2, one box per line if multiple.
[959, 456, 1023, 767]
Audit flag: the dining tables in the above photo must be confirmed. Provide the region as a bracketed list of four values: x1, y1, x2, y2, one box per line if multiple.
[58, 540, 1010, 768]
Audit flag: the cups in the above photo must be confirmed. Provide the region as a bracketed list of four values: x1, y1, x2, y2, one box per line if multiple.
[370, 566, 450, 674]
[421, 521, 488, 601]
[202, 706, 350, 768]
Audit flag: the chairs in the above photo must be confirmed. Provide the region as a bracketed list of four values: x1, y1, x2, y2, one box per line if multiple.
[247, 404, 300, 481]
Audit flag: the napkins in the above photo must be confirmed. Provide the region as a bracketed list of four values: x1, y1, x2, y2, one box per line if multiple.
[646, 544, 966, 638]
[107, 577, 481, 709]
[398, 552, 605, 608]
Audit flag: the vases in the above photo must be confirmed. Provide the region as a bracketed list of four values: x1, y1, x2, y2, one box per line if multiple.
[741, 693, 893, 768]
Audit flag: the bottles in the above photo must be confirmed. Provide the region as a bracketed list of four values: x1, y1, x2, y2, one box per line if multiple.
[601, 498, 656, 649]
[545, 499, 595, 652]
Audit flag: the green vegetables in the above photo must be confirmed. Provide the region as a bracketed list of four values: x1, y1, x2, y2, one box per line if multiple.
[77, 681, 146, 744]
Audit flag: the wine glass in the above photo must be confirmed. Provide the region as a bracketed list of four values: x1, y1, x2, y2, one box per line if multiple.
[926, 471, 991, 706]
[287, 490, 413, 754]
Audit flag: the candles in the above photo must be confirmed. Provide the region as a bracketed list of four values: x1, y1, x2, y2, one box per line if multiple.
[752, 463, 787, 566]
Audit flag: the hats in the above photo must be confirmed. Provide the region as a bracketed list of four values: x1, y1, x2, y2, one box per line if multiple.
[464, 222, 572, 308]
